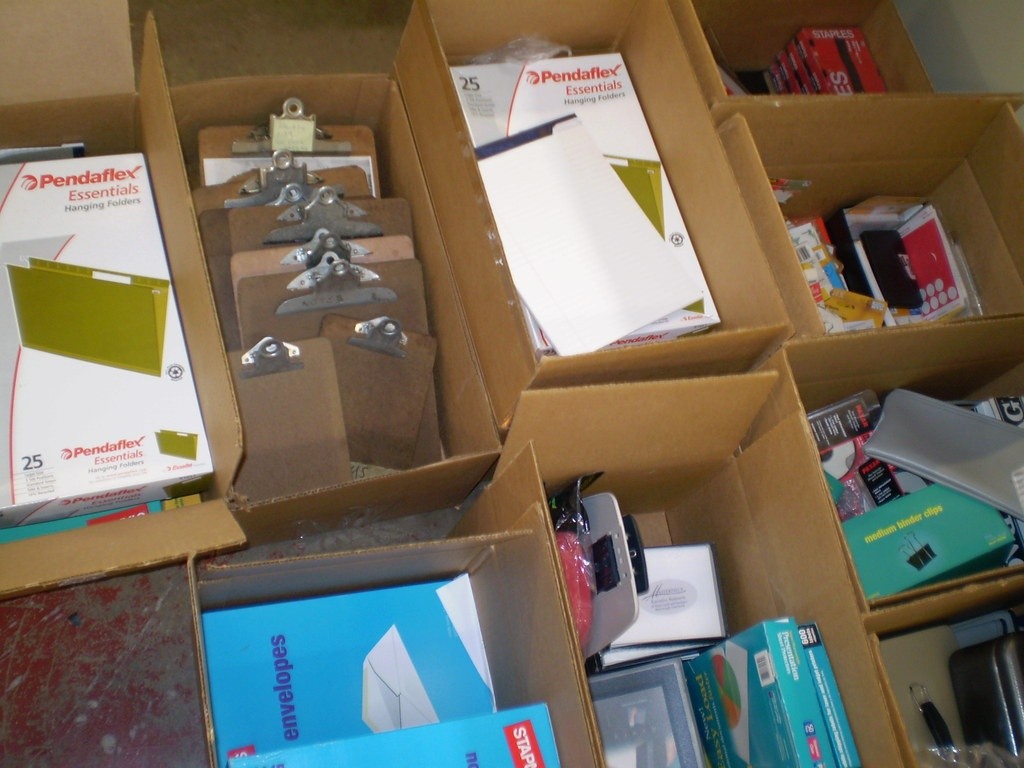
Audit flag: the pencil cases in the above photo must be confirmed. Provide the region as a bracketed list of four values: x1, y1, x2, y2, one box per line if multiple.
[862, 387, 1024, 521]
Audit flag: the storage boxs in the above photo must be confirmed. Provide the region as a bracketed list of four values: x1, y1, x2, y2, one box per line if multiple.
[0, 152, 215, 529]
[187, 501, 599, 768]
[682, 617, 837, 768]
[225, 702, 561, 768]
[0, 91, 248, 600]
[733, 314, 1024, 768]
[127, 0, 502, 546]
[445, 372, 905, 768]
[449, 51, 721, 362]
[797, 619, 863, 768]
[712, 97, 1024, 367]
[842, 481, 1015, 602]
[668, 0, 936, 109]
[767, 25, 887, 96]
[389, 0, 796, 443]
[201, 580, 493, 768]
